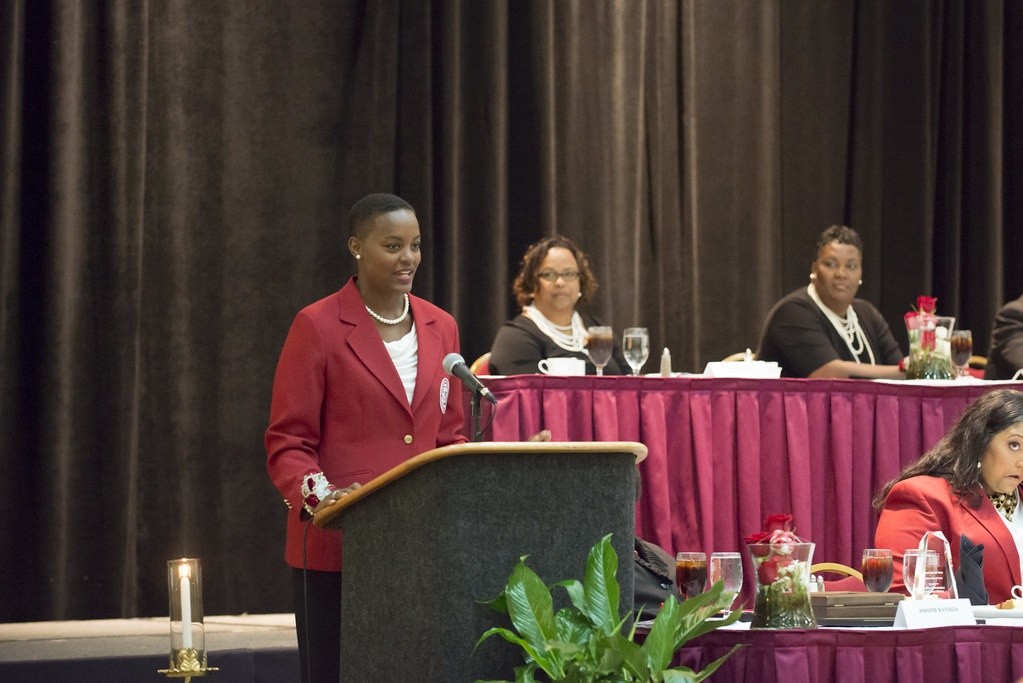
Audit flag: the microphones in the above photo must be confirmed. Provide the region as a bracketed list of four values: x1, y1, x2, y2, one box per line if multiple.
[442, 353, 496, 405]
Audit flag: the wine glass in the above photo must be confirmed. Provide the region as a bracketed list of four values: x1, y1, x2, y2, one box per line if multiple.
[951, 329, 971, 378]
[586, 326, 614, 374]
[623, 328, 650, 377]
[710, 553, 742, 621]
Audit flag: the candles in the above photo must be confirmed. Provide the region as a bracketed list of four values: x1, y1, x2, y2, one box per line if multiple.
[179, 562, 192, 651]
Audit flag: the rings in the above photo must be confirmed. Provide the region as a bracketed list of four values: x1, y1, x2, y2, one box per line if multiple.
[332, 490, 339, 499]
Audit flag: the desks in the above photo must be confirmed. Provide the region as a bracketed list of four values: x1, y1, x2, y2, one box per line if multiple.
[632, 605, 1023, 683]
[459, 374, 1023, 612]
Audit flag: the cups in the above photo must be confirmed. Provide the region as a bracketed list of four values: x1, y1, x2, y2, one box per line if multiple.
[676, 551, 708, 599]
[903, 548, 938, 596]
[539, 357, 585, 376]
[861, 549, 893, 592]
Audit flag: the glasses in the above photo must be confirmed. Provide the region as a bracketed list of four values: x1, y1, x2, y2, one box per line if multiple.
[537, 267, 581, 282]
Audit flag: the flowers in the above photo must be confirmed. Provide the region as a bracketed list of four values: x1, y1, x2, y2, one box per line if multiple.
[904, 295, 952, 374]
[741, 513, 814, 626]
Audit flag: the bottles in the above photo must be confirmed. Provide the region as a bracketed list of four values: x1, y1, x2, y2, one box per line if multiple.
[817, 575, 825, 591]
[809, 574, 818, 592]
[660, 346, 672, 378]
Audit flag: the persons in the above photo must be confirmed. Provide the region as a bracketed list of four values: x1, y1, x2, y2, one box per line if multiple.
[488, 234, 645, 377]
[756, 225, 911, 380]
[983, 293, 1023, 381]
[874, 384, 1022, 605]
[265, 193, 552, 683]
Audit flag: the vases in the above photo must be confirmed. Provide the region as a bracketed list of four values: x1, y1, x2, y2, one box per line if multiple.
[747, 543, 819, 629]
[903, 317, 957, 380]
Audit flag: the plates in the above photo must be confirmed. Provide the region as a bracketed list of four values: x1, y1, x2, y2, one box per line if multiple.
[972, 605, 1022, 618]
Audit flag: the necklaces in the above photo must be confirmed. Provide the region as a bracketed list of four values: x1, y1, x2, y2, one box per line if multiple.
[365, 293, 409, 324]
[807, 282, 876, 366]
[984, 490, 1017, 522]
[522, 304, 589, 352]
